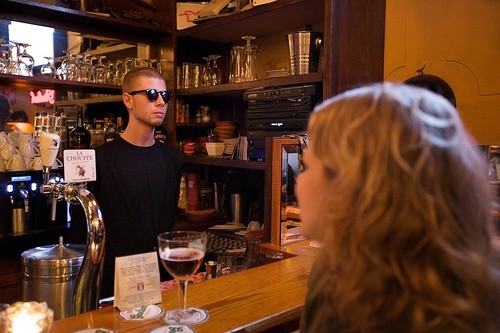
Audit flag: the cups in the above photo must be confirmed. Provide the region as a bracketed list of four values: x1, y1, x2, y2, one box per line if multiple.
[0, 303, 54, 333]
[0, 129, 64, 172]
[229, 36, 264, 85]
[247, 221, 261, 232]
[205, 260, 216, 278]
[175, 62, 202, 90]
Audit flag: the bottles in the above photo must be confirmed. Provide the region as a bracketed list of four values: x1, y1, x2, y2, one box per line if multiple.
[230, 193, 245, 225]
[85, 117, 123, 148]
[67, 107, 91, 149]
[22, 235, 87, 321]
[33, 110, 68, 158]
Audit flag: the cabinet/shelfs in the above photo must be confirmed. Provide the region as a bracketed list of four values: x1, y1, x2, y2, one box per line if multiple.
[0, 0, 385, 288]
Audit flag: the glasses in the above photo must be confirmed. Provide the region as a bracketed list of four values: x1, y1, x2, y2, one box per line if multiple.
[128, 88, 173, 104]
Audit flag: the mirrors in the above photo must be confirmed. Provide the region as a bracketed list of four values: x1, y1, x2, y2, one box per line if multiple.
[271, 137, 313, 248]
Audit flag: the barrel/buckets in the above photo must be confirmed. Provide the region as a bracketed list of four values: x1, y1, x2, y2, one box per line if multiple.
[285, 30, 323, 76]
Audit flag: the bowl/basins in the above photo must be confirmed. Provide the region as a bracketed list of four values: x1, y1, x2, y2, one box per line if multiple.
[206, 142, 225, 156]
[215, 120, 236, 143]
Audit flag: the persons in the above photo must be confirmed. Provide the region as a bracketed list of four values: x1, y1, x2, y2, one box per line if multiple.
[286, 81, 500, 333]
[0, 95, 11, 134]
[89, 67, 187, 301]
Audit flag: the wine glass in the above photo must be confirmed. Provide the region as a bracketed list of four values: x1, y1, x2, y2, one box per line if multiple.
[0, 38, 34, 77]
[156, 231, 209, 326]
[40, 49, 158, 87]
[200, 54, 222, 87]
